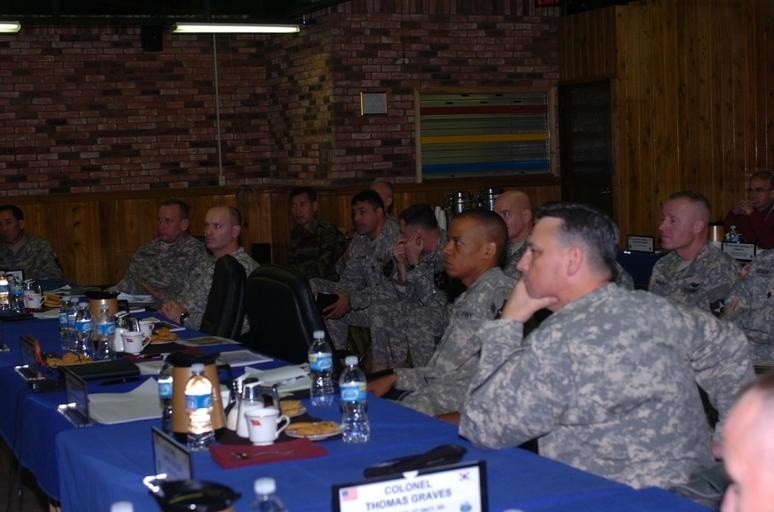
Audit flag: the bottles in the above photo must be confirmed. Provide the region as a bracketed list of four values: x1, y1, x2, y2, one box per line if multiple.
[0, 270, 10, 311]
[727, 223, 739, 245]
[339, 356, 371, 444]
[9, 272, 25, 315]
[308, 331, 334, 407]
[246, 477, 284, 512]
[74, 303, 95, 361]
[157, 364, 175, 439]
[59, 297, 72, 350]
[183, 363, 215, 452]
[227, 378, 250, 432]
[96, 304, 116, 360]
[68, 298, 80, 354]
[237, 385, 264, 439]
[111, 315, 130, 352]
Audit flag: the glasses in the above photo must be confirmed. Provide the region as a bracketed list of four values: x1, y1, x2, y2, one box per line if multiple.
[747, 187, 772, 193]
[203, 222, 235, 232]
[352, 208, 376, 216]
[158, 217, 182, 224]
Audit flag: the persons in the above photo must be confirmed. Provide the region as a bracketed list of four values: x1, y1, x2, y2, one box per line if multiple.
[494, 190, 534, 282]
[318, 188, 403, 353]
[104, 198, 211, 312]
[724, 172, 773, 246]
[718, 377, 774, 512]
[724, 248, 773, 367]
[306, 181, 398, 300]
[366, 208, 520, 427]
[160, 204, 260, 335]
[0, 205, 63, 281]
[457, 206, 754, 489]
[365, 207, 468, 375]
[649, 192, 741, 322]
[279, 189, 347, 281]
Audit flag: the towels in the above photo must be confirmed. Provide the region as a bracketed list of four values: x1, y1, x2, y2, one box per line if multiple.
[208, 437, 327, 468]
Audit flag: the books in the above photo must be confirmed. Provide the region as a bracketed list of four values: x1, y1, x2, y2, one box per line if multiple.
[315, 290, 340, 313]
[244, 362, 311, 392]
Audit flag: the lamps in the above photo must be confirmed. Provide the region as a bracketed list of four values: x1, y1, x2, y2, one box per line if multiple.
[168, 20, 307, 34]
[0, 20, 27, 34]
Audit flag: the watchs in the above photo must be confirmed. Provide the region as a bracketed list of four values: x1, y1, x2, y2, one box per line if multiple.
[179, 310, 190, 325]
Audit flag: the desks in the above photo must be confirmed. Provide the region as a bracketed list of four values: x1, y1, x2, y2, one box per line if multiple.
[0, 280, 715, 512]
[614, 247, 669, 291]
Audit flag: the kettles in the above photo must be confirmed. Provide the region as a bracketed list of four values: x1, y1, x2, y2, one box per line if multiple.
[159, 350, 236, 449]
[83, 289, 118, 343]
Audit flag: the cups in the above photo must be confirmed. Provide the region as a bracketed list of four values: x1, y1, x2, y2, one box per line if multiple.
[139, 321, 156, 339]
[122, 332, 150, 356]
[27, 293, 47, 310]
[238, 377, 280, 442]
[111, 317, 140, 354]
[249, 410, 291, 447]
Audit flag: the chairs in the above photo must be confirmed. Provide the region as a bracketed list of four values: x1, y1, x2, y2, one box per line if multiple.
[245, 262, 350, 383]
[383, 304, 556, 457]
[198, 254, 247, 342]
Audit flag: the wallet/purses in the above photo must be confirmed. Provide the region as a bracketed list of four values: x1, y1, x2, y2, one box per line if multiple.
[316, 292, 345, 317]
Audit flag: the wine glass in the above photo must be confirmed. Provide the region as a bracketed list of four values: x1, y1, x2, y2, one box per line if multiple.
[9, 280, 25, 315]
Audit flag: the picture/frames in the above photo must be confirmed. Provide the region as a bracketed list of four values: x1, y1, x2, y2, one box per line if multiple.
[360, 90, 388, 115]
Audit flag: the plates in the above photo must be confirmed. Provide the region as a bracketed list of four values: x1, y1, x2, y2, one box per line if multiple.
[145, 334, 180, 346]
[281, 401, 307, 420]
[285, 422, 343, 443]
